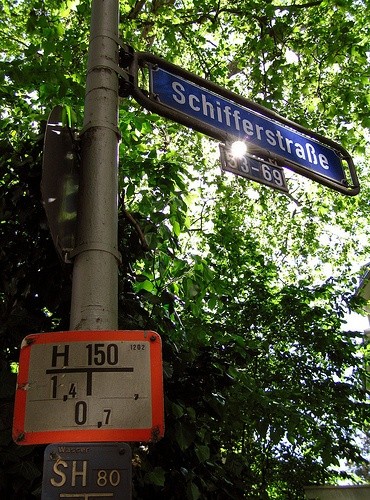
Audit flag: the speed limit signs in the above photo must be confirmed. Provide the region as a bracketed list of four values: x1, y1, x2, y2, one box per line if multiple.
[40, 442, 132, 500]
[11, 329, 167, 446]
